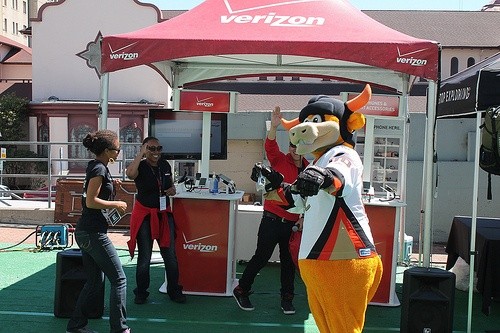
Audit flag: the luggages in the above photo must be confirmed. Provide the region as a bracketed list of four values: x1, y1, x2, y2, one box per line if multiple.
[53, 248, 105, 319]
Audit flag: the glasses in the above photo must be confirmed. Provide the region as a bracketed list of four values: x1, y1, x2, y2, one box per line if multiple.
[147, 145, 164, 151]
[108, 148, 121, 154]
[290, 142, 296, 148]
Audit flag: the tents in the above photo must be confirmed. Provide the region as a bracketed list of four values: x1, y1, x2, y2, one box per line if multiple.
[99, 0, 441, 268]
[420, 51, 500, 333]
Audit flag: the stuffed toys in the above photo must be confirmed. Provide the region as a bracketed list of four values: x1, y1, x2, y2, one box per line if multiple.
[280, 83, 383, 333]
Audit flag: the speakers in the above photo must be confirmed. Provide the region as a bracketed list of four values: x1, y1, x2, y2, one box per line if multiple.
[400, 266, 456, 333]
[54, 248, 105, 318]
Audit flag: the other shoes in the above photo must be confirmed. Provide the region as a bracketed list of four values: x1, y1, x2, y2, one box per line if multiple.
[167, 285, 186, 303]
[66, 326, 100, 333]
[133, 288, 150, 304]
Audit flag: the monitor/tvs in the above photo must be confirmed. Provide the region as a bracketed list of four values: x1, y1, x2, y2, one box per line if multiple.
[148, 108, 227, 160]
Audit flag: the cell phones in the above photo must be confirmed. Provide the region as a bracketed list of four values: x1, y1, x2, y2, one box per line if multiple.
[178, 172, 232, 185]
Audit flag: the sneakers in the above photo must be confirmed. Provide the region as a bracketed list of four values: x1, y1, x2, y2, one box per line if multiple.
[280, 303, 296, 314]
[232, 285, 255, 311]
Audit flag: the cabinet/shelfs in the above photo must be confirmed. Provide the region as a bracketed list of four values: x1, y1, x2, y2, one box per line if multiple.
[354, 114, 406, 198]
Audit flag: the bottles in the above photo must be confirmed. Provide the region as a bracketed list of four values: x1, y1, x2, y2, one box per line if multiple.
[209, 172, 218, 195]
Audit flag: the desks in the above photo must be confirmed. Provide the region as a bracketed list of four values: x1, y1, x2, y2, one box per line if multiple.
[444, 217, 500, 317]
[159, 189, 245, 296]
[357, 196, 408, 307]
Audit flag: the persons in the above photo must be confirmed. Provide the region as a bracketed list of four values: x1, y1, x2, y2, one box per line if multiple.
[233, 106, 310, 315]
[126, 136, 187, 304]
[66, 129, 131, 333]
